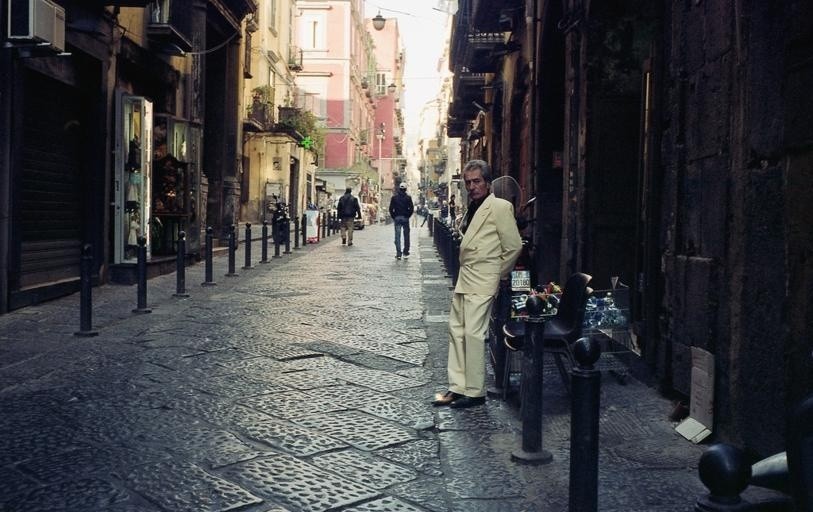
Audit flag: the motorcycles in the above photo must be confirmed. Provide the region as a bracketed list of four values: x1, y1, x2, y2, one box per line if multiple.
[269, 193, 291, 243]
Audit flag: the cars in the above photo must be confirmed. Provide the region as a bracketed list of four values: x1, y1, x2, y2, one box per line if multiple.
[327, 207, 365, 230]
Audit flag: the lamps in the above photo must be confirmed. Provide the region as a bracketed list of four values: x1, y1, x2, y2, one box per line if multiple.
[372, 9, 386, 30]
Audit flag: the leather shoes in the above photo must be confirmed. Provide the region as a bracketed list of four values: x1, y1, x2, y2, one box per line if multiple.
[431, 391, 485, 408]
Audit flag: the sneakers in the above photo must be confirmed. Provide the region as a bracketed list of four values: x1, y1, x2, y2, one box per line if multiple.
[397, 252, 410, 259]
[342, 237, 353, 246]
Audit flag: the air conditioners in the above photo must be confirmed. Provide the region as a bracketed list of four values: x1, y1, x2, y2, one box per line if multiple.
[0, 0, 72, 59]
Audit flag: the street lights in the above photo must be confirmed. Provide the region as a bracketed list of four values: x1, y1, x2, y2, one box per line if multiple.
[375, 134, 384, 204]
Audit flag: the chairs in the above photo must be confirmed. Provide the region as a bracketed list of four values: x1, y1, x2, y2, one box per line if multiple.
[501, 272, 593, 400]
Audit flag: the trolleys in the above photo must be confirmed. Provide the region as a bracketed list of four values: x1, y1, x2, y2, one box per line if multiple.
[502, 276, 634, 389]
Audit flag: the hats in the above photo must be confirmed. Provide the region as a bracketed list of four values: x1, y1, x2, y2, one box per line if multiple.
[346, 186, 351, 191]
[399, 183, 407, 189]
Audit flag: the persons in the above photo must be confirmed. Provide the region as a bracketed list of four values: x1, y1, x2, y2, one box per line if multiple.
[450, 194, 456, 227]
[413, 204, 430, 227]
[389, 183, 414, 259]
[441, 200, 448, 223]
[338, 188, 361, 246]
[431, 160, 522, 408]
